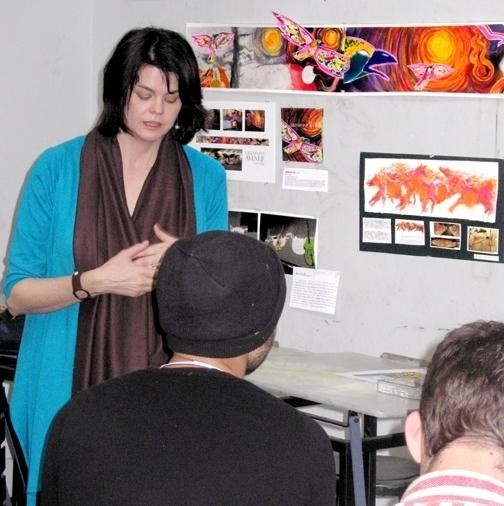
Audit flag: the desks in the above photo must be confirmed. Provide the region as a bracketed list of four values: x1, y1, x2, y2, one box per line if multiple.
[243, 349, 431, 506]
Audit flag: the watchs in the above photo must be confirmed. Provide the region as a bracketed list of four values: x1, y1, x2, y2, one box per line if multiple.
[71, 270, 92, 303]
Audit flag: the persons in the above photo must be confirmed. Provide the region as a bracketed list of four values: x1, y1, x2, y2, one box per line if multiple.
[38, 229, 338, 506]
[393, 319, 503, 506]
[1, 25, 230, 506]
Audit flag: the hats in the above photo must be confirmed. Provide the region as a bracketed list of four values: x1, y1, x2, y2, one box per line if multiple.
[154, 230, 286, 357]
